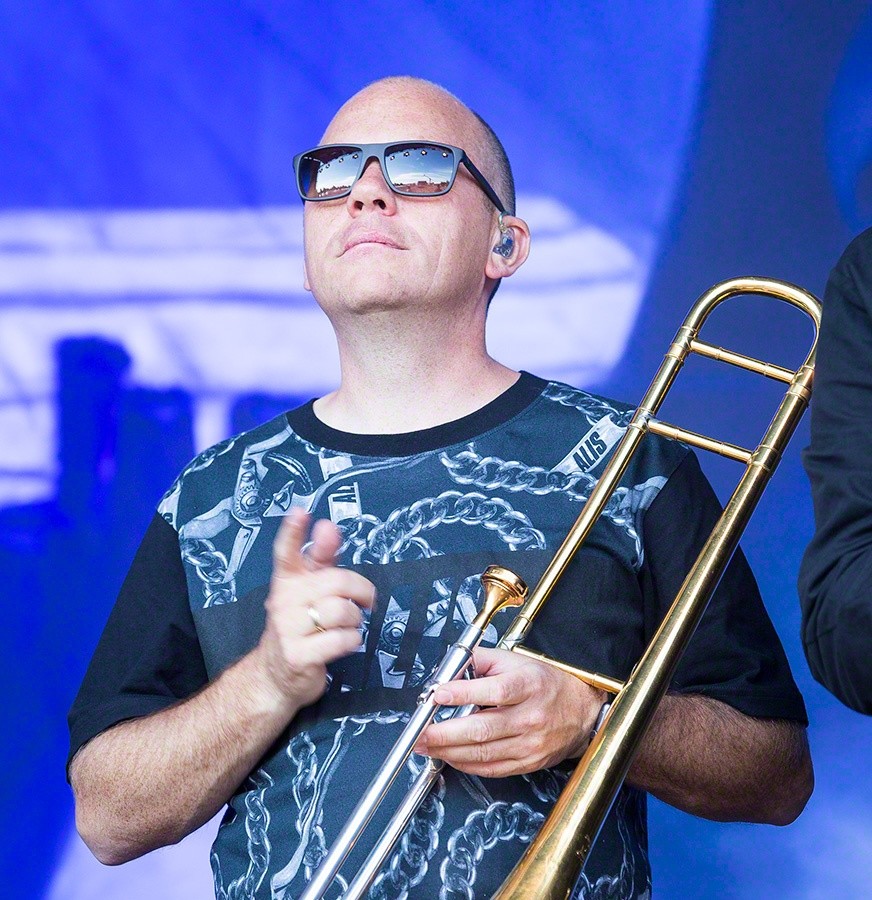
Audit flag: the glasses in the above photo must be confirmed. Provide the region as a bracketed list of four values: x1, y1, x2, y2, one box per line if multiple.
[292, 140, 506, 213]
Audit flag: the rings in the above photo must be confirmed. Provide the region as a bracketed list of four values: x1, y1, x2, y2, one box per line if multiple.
[302, 606, 331, 635]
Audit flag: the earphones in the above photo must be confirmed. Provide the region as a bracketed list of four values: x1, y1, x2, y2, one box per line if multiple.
[491, 225, 518, 261]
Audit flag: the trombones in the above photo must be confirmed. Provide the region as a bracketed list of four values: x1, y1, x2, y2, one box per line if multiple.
[298, 275, 824, 900]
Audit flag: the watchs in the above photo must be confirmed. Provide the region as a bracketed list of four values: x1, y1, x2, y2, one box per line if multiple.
[587, 695, 616, 745]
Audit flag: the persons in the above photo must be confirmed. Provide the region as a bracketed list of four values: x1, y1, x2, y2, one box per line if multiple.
[67, 76, 815, 900]
[798, 227, 871, 718]
